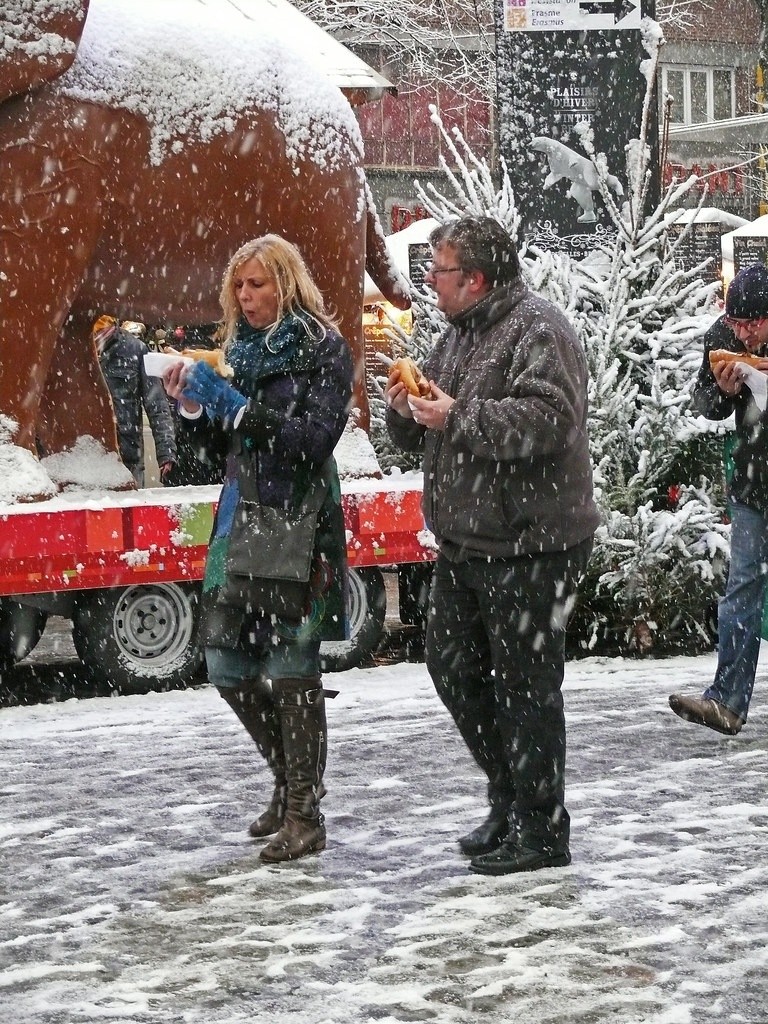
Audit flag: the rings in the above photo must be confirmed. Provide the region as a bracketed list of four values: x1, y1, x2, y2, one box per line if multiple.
[177, 386, 182, 389]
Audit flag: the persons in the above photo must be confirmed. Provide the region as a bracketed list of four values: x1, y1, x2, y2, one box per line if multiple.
[93, 314, 230, 488]
[164, 233, 357, 860]
[669, 261, 768, 735]
[386, 217, 600, 877]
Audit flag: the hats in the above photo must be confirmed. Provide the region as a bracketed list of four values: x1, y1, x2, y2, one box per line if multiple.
[726, 263, 768, 319]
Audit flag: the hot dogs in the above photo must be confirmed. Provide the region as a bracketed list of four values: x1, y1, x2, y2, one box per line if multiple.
[709, 349, 762, 372]
[387, 357, 431, 398]
[163, 345, 235, 378]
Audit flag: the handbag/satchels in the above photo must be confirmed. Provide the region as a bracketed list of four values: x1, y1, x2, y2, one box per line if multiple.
[217, 499, 318, 620]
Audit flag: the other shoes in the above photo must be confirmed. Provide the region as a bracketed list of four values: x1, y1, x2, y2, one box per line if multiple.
[457, 816, 510, 855]
[669, 694, 742, 736]
[468, 842, 572, 876]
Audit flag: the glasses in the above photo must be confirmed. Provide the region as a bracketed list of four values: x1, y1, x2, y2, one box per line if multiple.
[723, 316, 766, 329]
[432, 267, 472, 280]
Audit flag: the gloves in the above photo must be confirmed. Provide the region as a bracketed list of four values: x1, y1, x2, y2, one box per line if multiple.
[181, 361, 246, 424]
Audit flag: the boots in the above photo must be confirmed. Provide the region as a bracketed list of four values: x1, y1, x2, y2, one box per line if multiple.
[216, 674, 287, 837]
[259, 678, 340, 863]
